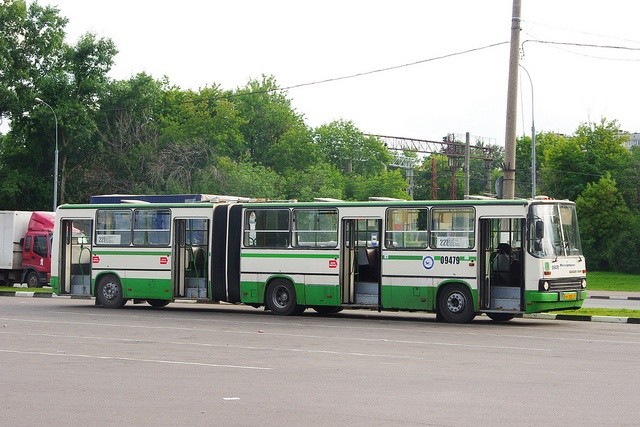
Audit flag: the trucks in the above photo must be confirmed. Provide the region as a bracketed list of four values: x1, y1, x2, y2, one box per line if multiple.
[0, 210, 88, 287]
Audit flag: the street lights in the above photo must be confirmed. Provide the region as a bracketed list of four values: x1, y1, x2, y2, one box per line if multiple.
[34, 97, 59, 211]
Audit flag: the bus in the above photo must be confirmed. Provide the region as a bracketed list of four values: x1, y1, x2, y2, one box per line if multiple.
[50, 195, 586, 322]
